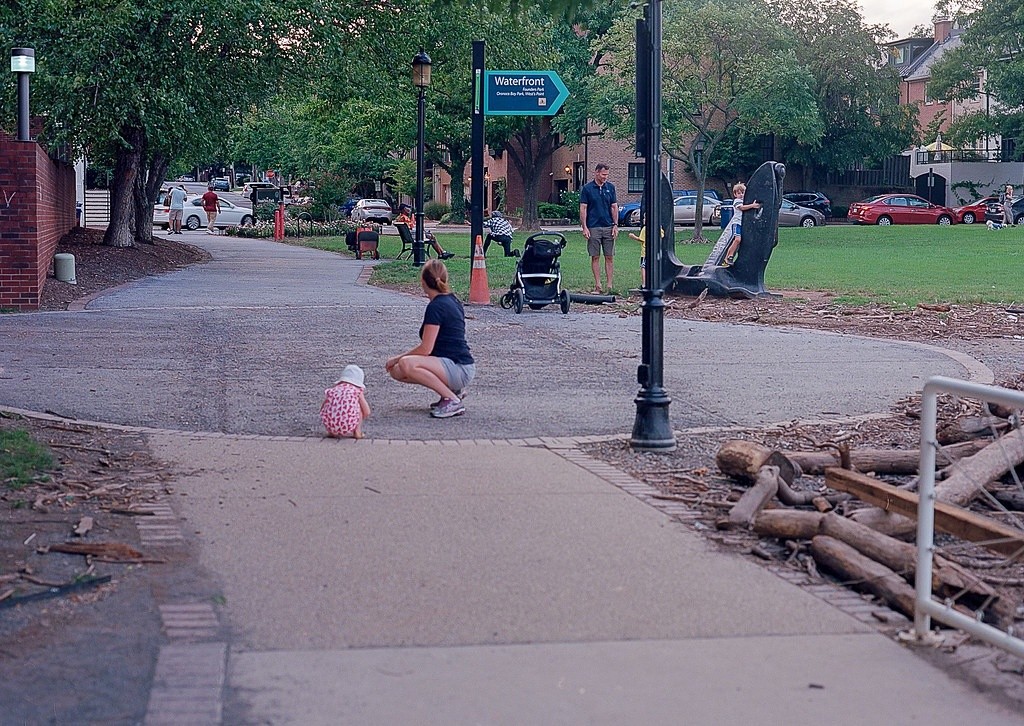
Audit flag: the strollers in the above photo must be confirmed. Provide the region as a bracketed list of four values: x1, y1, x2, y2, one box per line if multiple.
[500, 231, 571, 314]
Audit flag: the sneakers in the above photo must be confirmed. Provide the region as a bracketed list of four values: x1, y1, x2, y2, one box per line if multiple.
[430, 398, 466, 418]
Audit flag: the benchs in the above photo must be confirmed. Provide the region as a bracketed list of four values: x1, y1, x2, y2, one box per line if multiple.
[392, 222, 438, 262]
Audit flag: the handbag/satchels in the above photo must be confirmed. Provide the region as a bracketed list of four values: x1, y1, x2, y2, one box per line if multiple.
[164, 197, 168, 206]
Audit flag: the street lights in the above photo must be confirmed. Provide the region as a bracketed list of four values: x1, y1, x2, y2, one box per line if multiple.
[408, 46, 435, 267]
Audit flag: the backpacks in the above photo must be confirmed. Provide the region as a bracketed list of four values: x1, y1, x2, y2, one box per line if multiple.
[999, 192, 1009, 204]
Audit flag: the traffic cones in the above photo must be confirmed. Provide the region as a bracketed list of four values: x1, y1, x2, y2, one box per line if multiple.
[462, 234, 497, 307]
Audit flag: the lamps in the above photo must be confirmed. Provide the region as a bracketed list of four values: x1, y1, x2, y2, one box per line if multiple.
[467, 176, 472, 182]
[485, 172, 490, 179]
[565, 164, 572, 176]
[436, 174, 441, 183]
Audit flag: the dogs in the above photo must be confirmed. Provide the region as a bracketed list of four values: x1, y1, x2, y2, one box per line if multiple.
[985, 219, 1007, 231]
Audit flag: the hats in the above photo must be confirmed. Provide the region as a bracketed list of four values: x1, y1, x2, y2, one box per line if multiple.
[334, 364, 365, 388]
[399, 203, 411, 210]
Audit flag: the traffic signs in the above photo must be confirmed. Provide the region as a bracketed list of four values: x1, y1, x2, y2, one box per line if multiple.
[484, 70, 572, 117]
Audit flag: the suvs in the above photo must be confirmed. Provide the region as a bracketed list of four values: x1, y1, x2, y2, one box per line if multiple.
[782, 191, 833, 219]
[242, 181, 280, 202]
[618, 189, 720, 227]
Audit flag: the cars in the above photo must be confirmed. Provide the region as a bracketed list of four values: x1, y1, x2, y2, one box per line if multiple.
[983, 194, 1024, 226]
[153, 194, 257, 231]
[952, 196, 1002, 224]
[174, 174, 196, 182]
[712, 198, 826, 227]
[339, 198, 393, 226]
[207, 172, 253, 192]
[847, 193, 958, 226]
[630, 195, 724, 226]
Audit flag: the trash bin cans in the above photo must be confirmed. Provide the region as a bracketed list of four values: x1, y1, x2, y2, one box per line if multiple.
[720, 199, 734, 230]
[76, 209, 82, 227]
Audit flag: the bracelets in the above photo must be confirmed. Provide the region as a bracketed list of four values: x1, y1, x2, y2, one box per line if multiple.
[613, 223, 618, 225]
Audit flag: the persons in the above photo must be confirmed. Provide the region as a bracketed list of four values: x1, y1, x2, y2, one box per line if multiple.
[167, 184, 187, 235]
[387, 259, 476, 418]
[483, 209, 521, 258]
[202, 186, 221, 235]
[629, 213, 664, 290]
[724, 182, 760, 265]
[320, 364, 371, 441]
[207, 174, 215, 185]
[1002, 185, 1016, 228]
[394, 203, 456, 259]
[578, 163, 618, 295]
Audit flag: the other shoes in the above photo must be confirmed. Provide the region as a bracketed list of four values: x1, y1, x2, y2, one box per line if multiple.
[168, 230, 173, 235]
[638, 285, 646, 290]
[174, 231, 182, 234]
[206, 230, 214, 235]
[514, 249, 521, 258]
[603, 287, 611, 295]
[442, 251, 455, 260]
[438, 254, 443, 259]
[591, 286, 602, 295]
[724, 255, 734, 266]
